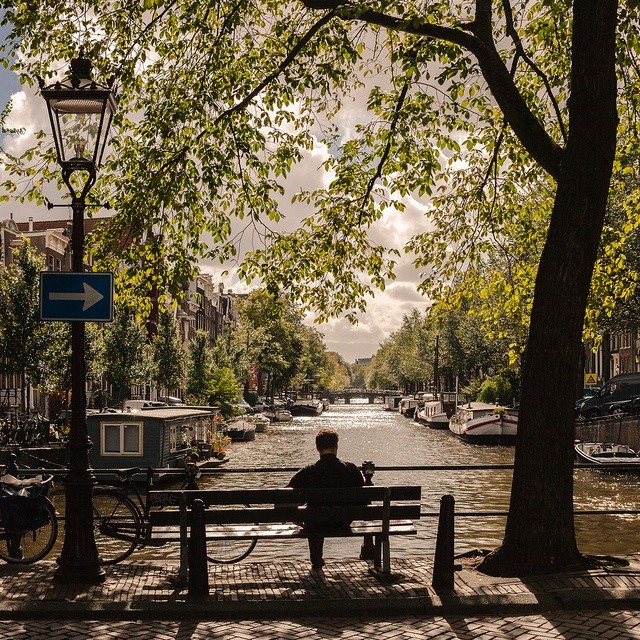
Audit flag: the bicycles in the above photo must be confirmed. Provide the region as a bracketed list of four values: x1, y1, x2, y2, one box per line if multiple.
[0, 464, 57, 564]
[88, 450, 259, 565]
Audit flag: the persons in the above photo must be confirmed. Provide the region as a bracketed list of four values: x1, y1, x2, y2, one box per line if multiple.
[284, 427, 364, 573]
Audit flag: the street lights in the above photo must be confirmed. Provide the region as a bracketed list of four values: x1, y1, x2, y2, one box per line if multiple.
[40, 46, 120, 586]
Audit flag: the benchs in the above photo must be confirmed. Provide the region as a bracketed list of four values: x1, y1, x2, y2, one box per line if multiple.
[146, 485, 421, 587]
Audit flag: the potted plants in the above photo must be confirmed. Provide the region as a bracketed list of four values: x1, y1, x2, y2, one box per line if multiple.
[189, 453, 199, 464]
[210, 432, 231, 459]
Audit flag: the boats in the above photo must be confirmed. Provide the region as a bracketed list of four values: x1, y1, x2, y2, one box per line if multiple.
[449, 401, 518, 446]
[417, 401, 450, 430]
[284, 398, 324, 415]
[219, 426, 256, 442]
[575, 439, 640, 465]
[274, 410, 293, 422]
[241, 415, 268, 432]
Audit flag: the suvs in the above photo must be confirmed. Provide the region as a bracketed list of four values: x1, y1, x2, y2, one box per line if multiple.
[580, 372, 640, 419]
